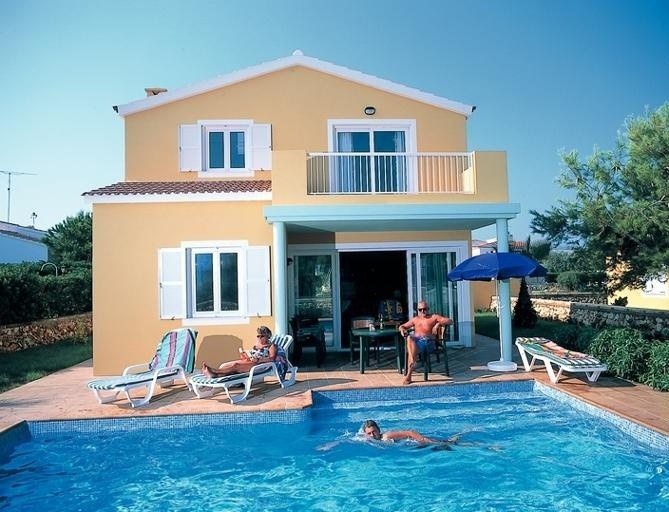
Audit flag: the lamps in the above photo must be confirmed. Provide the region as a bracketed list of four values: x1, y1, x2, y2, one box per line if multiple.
[365, 107, 376, 115]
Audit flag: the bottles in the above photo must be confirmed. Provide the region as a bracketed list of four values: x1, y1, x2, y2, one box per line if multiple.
[380, 317, 384, 329]
[369, 323, 376, 332]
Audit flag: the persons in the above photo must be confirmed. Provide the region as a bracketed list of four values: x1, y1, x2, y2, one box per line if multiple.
[202, 325, 279, 379]
[316, 419, 474, 451]
[398, 301, 453, 385]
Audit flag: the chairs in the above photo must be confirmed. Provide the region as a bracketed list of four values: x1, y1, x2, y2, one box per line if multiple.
[348, 300, 453, 381]
[87, 328, 198, 408]
[515, 337, 608, 384]
[186, 334, 298, 405]
[289, 316, 325, 368]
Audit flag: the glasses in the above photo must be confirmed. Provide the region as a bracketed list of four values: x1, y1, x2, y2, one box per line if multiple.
[257, 335, 265, 337]
[419, 308, 427, 312]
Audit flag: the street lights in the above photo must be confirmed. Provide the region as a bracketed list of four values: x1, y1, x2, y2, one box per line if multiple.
[29, 211, 38, 226]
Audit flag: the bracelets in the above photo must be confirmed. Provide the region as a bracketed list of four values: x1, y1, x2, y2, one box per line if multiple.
[256, 358, 259, 363]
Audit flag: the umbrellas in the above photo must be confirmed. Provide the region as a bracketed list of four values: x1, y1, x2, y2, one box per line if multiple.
[447, 252, 548, 357]
